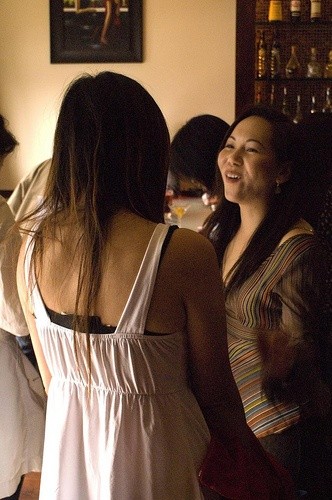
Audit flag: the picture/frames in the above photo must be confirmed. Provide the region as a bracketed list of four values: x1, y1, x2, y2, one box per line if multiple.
[49, 0, 144, 63]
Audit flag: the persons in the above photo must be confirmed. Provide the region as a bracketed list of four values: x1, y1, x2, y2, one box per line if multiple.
[197, 106, 324, 484]
[0, 113, 331, 500]
[4, 71, 252, 500]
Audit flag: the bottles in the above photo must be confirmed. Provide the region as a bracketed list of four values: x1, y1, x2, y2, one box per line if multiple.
[256, 84, 332, 124]
[256, 28, 332, 78]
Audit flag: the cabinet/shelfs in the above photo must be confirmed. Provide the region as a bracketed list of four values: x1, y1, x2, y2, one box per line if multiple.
[235, 0, 332, 124]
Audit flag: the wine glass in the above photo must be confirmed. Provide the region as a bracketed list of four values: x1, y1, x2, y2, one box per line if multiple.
[168, 201, 192, 228]
